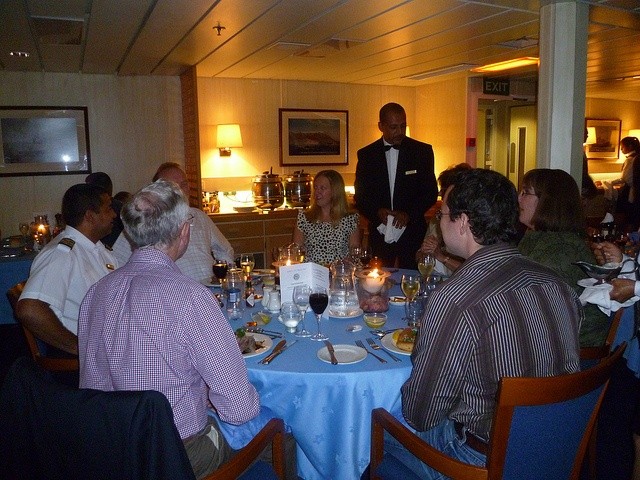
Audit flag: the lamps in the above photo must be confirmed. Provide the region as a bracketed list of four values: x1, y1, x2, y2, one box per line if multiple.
[584, 127, 597, 147]
[215, 124, 243, 156]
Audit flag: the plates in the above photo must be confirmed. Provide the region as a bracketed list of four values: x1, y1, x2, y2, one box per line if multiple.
[235, 332, 273, 358]
[389, 296, 406, 306]
[252, 268, 276, 277]
[380, 329, 420, 355]
[577, 275, 627, 288]
[242, 293, 264, 304]
[317, 344, 367, 365]
[201, 276, 222, 288]
[327, 304, 364, 318]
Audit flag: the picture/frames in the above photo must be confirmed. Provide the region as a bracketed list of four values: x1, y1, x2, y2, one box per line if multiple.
[1, 105, 92, 177]
[278, 107, 349, 166]
[584, 119, 622, 159]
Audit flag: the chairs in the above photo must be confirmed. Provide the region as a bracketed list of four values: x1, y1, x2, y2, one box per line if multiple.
[579, 306, 636, 371]
[34, 384, 297, 478]
[7, 279, 81, 385]
[368, 341, 630, 480]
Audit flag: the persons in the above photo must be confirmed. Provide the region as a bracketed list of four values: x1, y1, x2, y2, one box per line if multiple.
[381, 166, 586, 480]
[83, 172, 127, 248]
[577, 115, 600, 202]
[587, 225, 640, 480]
[509, 168, 611, 348]
[351, 101, 439, 273]
[10, 182, 120, 357]
[110, 162, 237, 287]
[75, 176, 263, 480]
[413, 161, 499, 278]
[293, 169, 363, 270]
[608, 135, 639, 237]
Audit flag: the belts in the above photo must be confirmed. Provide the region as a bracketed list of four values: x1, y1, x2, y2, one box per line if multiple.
[451, 422, 489, 456]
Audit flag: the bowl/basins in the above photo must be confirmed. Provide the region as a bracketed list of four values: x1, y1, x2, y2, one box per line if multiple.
[363, 313, 387, 327]
[251, 310, 274, 326]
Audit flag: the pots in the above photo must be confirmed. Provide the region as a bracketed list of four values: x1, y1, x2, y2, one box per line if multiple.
[284, 169, 313, 207]
[251, 166, 284, 208]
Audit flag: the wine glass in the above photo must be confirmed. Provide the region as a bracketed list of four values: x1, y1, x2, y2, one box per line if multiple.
[292, 284, 312, 337]
[401, 274, 420, 302]
[350, 243, 372, 271]
[281, 301, 300, 334]
[240, 253, 255, 277]
[309, 287, 329, 341]
[212, 259, 229, 299]
[418, 255, 433, 296]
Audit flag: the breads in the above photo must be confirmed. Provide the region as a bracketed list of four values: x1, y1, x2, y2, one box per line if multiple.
[391, 330, 401, 346]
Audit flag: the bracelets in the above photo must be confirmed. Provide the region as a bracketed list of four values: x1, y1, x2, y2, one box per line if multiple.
[442, 255, 452, 264]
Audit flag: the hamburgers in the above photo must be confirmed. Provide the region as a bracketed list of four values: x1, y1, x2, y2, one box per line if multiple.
[397, 327, 415, 350]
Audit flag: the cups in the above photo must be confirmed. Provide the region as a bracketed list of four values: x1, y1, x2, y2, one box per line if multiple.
[405, 294, 425, 327]
[570, 258, 638, 286]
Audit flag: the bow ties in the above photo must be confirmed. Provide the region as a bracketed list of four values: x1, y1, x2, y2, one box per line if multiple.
[383, 144, 401, 152]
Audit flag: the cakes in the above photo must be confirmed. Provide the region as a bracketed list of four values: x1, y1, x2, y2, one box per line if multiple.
[237, 336, 257, 353]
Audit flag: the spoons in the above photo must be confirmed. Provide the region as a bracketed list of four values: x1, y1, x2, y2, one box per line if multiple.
[257, 339, 287, 364]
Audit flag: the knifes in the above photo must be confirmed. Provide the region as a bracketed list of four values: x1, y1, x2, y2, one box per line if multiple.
[261, 340, 300, 364]
[324, 340, 338, 365]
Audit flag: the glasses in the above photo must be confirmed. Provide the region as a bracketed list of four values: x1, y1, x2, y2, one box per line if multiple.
[522, 189, 537, 198]
[179, 213, 195, 237]
[435, 210, 457, 222]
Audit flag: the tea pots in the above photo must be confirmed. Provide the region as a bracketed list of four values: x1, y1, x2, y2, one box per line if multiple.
[328, 260, 360, 317]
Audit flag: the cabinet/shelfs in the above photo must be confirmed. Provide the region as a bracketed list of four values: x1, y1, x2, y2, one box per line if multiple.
[208, 209, 298, 269]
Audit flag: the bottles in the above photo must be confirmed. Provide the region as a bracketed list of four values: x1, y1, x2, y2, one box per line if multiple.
[201, 191, 220, 215]
[261, 286, 273, 311]
[245, 276, 255, 307]
[268, 291, 281, 313]
[226, 263, 242, 320]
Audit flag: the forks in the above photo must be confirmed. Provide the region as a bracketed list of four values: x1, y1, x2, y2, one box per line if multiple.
[355, 339, 387, 364]
[366, 337, 402, 363]
